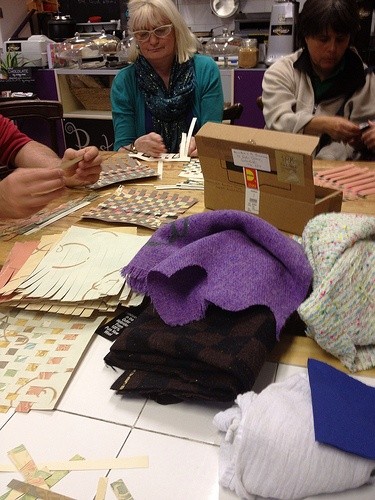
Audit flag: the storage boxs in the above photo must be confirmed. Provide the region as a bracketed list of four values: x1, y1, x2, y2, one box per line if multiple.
[195, 122, 343, 236]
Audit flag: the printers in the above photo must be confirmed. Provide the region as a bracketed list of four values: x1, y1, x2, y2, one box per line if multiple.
[3, 8, 59, 68]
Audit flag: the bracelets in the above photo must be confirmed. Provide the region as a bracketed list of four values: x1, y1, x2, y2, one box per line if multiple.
[131, 143, 138, 151]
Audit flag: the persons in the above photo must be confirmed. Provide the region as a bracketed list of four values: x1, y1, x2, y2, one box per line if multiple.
[111, 0, 224, 158]
[0, 114, 104, 222]
[262, 0, 375, 162]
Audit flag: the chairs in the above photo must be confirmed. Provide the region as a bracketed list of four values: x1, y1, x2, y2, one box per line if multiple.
[0, 102, 63, 180]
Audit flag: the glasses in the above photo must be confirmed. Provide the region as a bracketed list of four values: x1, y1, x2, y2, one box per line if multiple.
[128, 23, 174, 44]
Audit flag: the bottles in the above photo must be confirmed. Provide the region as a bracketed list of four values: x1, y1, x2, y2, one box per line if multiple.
[238, 39, 258, 69]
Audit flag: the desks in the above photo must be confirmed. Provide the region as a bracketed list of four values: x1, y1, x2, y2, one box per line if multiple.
[0, 151, 375, 500]
